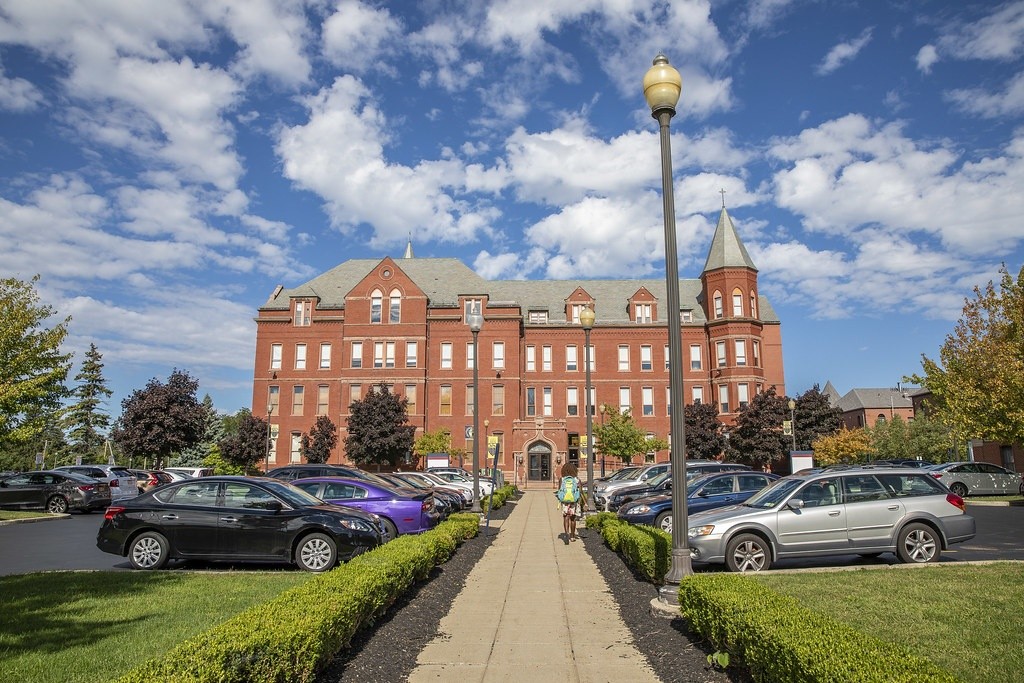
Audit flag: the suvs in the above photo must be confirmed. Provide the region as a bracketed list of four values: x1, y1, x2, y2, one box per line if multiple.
[1, 465, 213, 515]
[581, 459, 1024, 573]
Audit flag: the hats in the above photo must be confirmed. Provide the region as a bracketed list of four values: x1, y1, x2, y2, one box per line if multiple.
[561, 463, 578, 478]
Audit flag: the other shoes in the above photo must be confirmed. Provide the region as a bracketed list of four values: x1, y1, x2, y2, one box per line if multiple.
[571, 537, 576, 541]
[565, 533, 570, 545]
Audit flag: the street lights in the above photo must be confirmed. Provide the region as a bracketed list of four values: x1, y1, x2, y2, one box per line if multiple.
[129, 458, 133, 469]
[468, 305, 485, 521]
[484, 415, 490, 476]
[787, 398, 796, 450]
[641, 53, 695, 617]
[266, 404, 273, 473]
[598, 402, 605, 477]
[580, 303, 597, 519]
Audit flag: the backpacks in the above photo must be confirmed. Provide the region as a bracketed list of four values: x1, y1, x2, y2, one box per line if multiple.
[557, 476, 580, 503]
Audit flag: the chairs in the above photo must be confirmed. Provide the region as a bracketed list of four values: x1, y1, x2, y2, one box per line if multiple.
[35, 474, 47, 484]
[858, 484, 888, 500]
[224, 488, 253, 507]
[718, 481, 734, 494]
[329, 488, 347, 498]
[808, 483, 836, 507]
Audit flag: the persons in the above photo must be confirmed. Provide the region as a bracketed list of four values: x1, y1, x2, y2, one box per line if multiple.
[557, 463, 589, 544]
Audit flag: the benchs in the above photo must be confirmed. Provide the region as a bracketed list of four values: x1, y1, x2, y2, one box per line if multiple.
[178, 490, 216, 506]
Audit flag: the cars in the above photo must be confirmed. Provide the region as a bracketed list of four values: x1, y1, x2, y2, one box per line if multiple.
[96, 464, 497, 573]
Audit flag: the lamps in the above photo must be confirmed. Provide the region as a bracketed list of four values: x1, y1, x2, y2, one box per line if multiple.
[556, 454, 561, 464]
[717, 368, 721, 374]
[496, 370, 500, 374]
[270, 369, 276, 376]
[519, 455, 523, 463]
